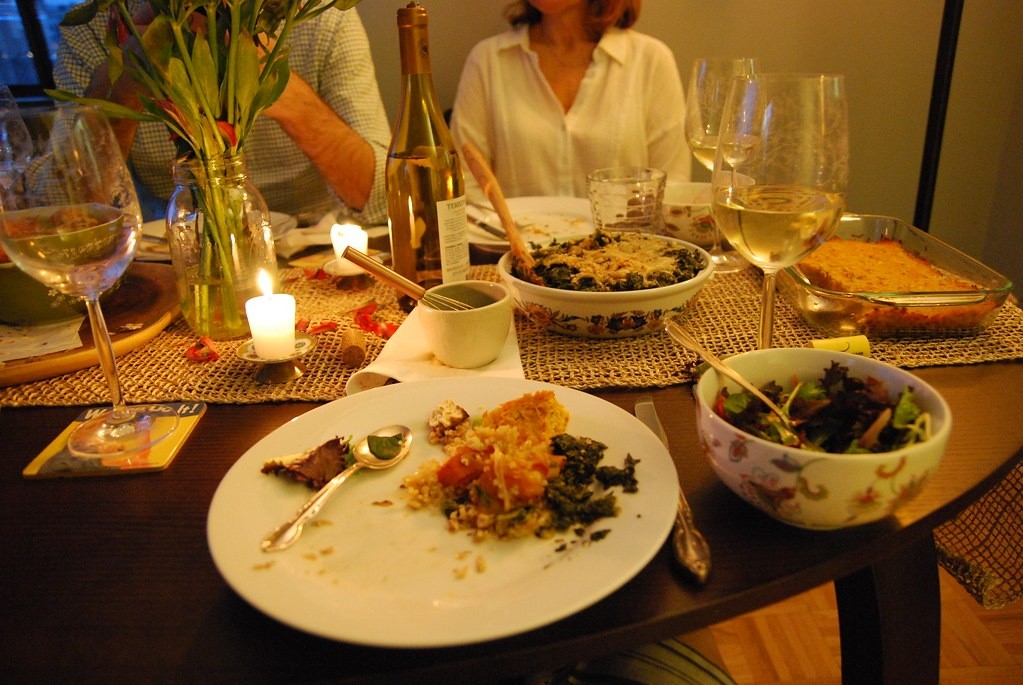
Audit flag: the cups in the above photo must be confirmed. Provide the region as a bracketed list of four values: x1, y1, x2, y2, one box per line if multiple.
[585, 166, 667, 237]
[417, 281, 511, 370]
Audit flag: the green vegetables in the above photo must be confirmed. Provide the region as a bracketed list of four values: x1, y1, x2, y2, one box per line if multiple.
[715, 360, 922, 452]
[509, 228, 706, 292]
[544, 433, 641, 553]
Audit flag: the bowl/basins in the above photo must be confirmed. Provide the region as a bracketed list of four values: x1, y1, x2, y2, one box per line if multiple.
[496, 236, 715, 339]
[0, 207, 124, 327]
[659, 181, 726, 250]
[693, 347, 953, 531]
[779, 212, 1014, 337]
[718, 171, 756, 189]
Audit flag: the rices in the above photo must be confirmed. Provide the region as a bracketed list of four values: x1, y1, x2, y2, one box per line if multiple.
[402, 423, 565, 579]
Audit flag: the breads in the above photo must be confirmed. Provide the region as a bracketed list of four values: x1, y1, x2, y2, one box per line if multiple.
[483, 390, 569, 445]
[798, 237, 994, 332]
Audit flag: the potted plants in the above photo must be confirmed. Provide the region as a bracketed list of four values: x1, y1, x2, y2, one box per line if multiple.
[52, 0, 353, 343]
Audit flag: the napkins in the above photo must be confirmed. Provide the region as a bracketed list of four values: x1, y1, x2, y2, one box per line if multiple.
[345, 308, 526, 397]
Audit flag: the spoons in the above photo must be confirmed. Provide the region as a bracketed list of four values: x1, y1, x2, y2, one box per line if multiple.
[260, 425, 413, 554]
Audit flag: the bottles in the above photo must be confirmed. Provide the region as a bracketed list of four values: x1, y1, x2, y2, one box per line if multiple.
[384, 5, 471, 314]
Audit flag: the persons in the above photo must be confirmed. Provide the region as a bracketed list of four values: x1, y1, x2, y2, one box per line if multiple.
[24, 0, 396, 226]
[446, 0, 692, 201]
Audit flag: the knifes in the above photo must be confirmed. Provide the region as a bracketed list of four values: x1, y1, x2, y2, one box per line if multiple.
[464, 214, 508, 240]
[635, 396, 711, 583]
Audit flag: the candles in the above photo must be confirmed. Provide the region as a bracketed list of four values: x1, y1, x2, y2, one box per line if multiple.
[244, 268, 296, 360]
[330, 223, 368, 267]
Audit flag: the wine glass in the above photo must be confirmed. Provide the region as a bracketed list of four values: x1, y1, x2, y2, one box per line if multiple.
[0, 105, 180, 461]
[692, 73, 849, 382]
[684, 57, 762, 274]
[1, 84, 33, 210]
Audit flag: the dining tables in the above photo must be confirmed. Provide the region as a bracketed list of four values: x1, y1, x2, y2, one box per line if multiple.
[0, 198, 1023, 685]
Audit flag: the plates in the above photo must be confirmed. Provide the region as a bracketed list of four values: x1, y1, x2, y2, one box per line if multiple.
[134, 209, 298, 262]
[205, 377, 679, 650]
[464, 196, 596, 253]
